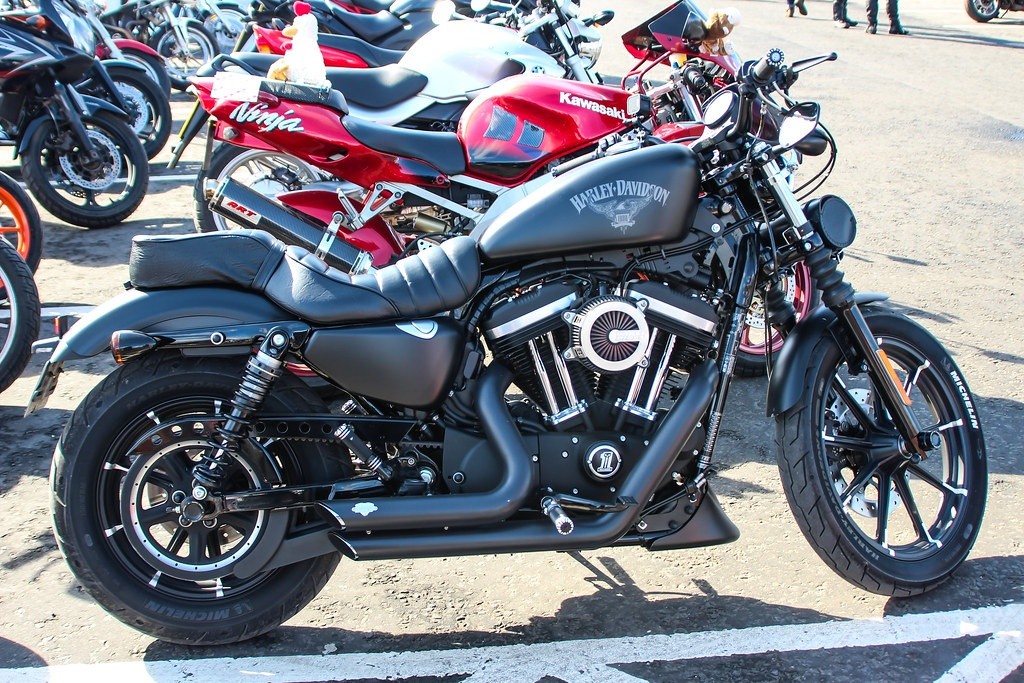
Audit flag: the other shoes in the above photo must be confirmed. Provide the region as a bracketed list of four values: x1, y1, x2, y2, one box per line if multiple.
[787, 7, 794, 16]
[845, 18, 859, 26]
[833, 20, 848, 28]
[866, 21, 878, 34]
[795, 2, 808, 15]
[889, 26, 909, 35]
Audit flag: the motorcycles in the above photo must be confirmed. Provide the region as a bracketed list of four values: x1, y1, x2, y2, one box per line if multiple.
[24, 49, 989, 648]
[965, 0, 1024, 23]
[1, 0, 822, 397]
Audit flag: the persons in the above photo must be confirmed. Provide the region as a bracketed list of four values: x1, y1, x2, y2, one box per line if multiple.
[865, 0, 909, 35]
[832, 0, 859, 28]
[786, 0, 808, 17]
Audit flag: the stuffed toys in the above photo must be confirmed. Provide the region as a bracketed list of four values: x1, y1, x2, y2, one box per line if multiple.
[266, 0, 332, 87]
[698, 8, 741, 57]
